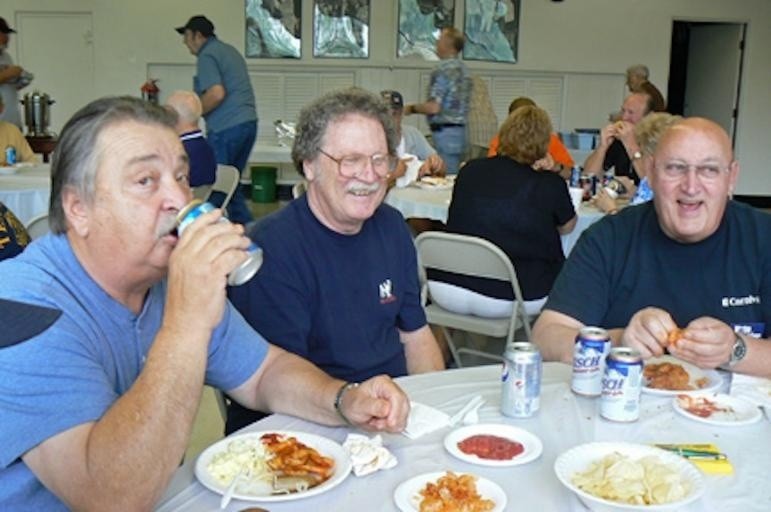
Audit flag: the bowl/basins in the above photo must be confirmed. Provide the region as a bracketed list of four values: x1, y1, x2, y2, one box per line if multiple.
[0, 164, 22, 175]
[551, 440, 708, 512]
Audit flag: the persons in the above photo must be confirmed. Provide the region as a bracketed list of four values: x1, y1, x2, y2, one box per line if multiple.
[581, 86, 656, 188]
[165, 88, 216, 191]
[379, 90, 447, 189]
[225, 88, 447, 433]
[529, 117, 771, 379]
[627, 65, 665, 114]
[402, 27, 472, 176]
[0, 101, 41, 168]
[0, 95, 409, 512]
[425, 105, 579, 320]
[486, 98, 575, 182]
[1, 19, 36, 134]
[623, 113, 684, 209]
[174, 16, 257, 225]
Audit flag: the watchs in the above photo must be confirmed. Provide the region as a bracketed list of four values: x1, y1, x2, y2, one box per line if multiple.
[631, 150, 643, 165]
[720, 330, 747, 369]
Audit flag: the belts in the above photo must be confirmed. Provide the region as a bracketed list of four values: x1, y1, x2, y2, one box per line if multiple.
[430, 122, 466, 131]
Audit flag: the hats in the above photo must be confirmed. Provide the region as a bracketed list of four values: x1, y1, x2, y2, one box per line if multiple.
[0, 17, 19, 35]
[173, 14, 216, 38]
[379, 89, 404, 111]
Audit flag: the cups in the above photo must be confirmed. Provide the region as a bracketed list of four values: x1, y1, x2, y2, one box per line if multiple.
[567, 185, 584, 211]
[606, 186, 617, 201]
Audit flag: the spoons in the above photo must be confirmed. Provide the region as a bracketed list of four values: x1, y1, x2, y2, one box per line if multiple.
[464, 398, 490, 422]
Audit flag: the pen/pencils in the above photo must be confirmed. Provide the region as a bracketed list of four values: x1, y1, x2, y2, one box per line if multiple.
[669, 449, 727, 460]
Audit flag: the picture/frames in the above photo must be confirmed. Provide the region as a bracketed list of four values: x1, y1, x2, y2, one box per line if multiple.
[312, 0, 371, 59]
[244, 1, 302, 59]
[395, 0, 456, 61]
[462, 0, 521, 65]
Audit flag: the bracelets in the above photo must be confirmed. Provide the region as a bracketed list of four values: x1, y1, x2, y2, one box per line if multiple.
[607, 208, 618, 216]
[386, 172, 391, 182]
[410, 104, 416, 114]
[335, 380, 359, 430]
[557, 163, 563, 174]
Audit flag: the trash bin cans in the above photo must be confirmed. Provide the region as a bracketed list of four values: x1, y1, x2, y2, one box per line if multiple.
[252, 166, 277, 204]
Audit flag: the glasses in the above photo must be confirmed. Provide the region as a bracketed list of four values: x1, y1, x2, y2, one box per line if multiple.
[316, 146, 398, 182]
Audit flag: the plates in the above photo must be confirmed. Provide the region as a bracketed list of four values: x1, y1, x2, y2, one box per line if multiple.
[446, 427, 543, 467]
[638, 356, 727, 398]
[672, 392, 761, 426]
[394, 471, 509, 512]
[411, 180, 454, 192]
[194, 429, 353, 503]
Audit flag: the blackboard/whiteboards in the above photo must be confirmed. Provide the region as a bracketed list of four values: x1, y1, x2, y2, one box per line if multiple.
[146, 63, 627, 162]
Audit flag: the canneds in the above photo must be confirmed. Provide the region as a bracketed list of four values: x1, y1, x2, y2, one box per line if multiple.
[175, 198, 264, 286]
[599, 348, 643, 423]
[570, 326, 611, 396]
[570, 166, 614, 201]
[500, 342, 542, 417]
[5, 146, 16, 166]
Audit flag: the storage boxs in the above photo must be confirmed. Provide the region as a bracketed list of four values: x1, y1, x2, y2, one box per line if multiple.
[572, 132, 601, 151]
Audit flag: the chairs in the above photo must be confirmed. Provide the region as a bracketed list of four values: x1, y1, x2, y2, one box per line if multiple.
[206, 162, 244, 217]
[412, 230, 537, 368]
